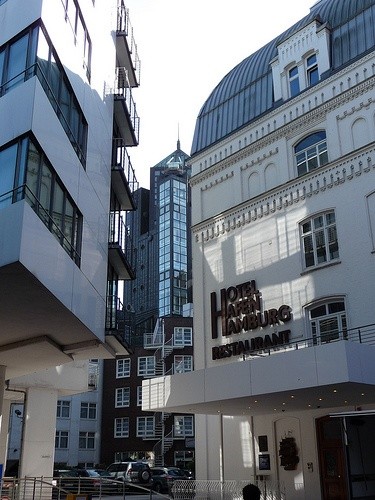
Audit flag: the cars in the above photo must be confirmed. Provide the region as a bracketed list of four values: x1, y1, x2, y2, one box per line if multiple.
[60, 466, 115, 494]
[54, 469, 70, 481]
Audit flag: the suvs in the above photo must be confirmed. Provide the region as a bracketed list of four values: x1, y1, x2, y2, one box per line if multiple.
[104, 462, 151, 493]
[150, 466, 189, 493]
